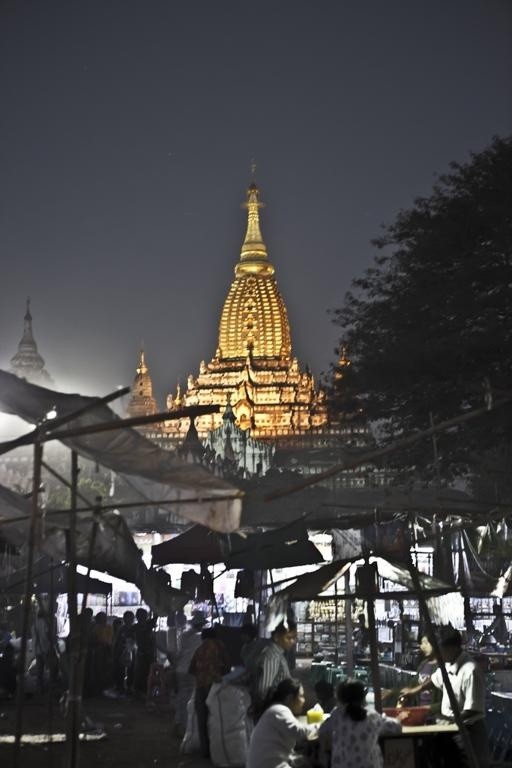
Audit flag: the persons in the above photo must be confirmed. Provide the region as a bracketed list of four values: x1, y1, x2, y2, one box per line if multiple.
[31, 602, 208, 711]
[181, 617, 402, 766]
[380, 624, 489, 768]
[482, 604, 507, 646]
[352, 614, 370, 658]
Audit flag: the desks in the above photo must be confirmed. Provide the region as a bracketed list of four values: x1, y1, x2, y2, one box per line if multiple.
[295, 687, 460, 767]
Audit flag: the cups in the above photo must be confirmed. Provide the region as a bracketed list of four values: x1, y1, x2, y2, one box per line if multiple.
[306, 708, 325, 724]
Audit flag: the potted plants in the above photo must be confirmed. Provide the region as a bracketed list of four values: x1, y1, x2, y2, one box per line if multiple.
[382, 658, 431, 725]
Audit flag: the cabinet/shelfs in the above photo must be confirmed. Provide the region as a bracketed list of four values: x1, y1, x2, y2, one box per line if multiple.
[292, 596, 368, 664]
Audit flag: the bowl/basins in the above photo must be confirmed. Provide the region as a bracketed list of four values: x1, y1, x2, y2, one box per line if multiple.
[382, 705, 431, 726]
